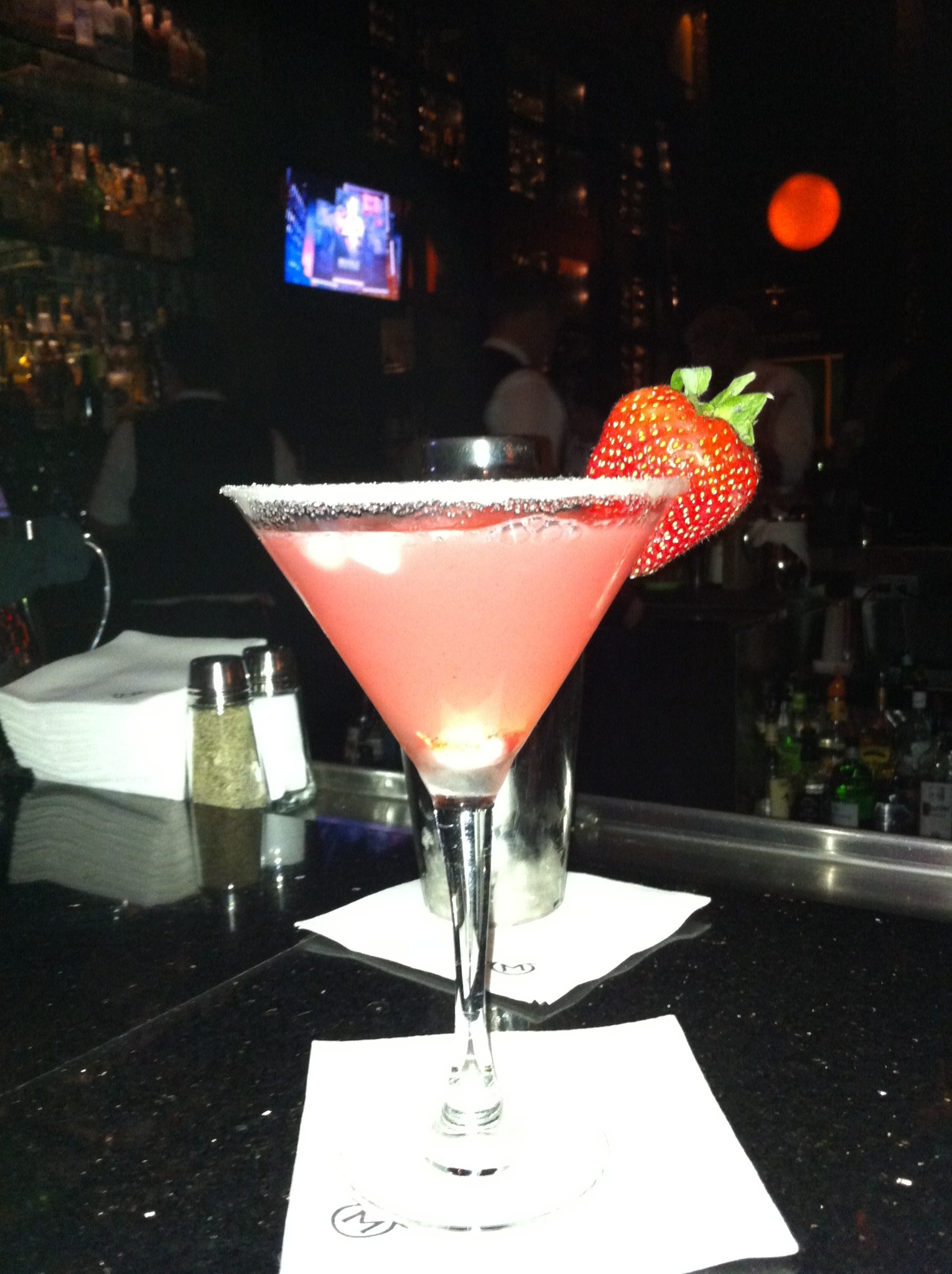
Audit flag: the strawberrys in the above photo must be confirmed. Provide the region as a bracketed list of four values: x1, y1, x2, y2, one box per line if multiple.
[585, 366, 759, 578]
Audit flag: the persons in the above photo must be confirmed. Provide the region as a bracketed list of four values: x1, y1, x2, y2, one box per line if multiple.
[92, 313, 309, 645]
[341, 303, 818, 627]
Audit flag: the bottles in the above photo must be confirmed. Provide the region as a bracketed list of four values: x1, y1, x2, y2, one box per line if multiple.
[0, 514, 109, 685]
[757, 680, 874, 828]
[860, 655, 952, 841]
[0, 288, 63, 423]
[188, 654, 272, 809]
[0, 0, 208, 98]
[0, 118, 75, 244]
[239, 641, 319, 815]
[402, 435, 575, 929]
[343, 695, 394, 769]
[112, 298, 161, 414]
[71, 133, 149, 256]
[60, 289, 112, 427]
[149, 163, 195, 262]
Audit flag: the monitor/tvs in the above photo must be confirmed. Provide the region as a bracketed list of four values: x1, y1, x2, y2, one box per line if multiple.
[273, 166, 409, 309]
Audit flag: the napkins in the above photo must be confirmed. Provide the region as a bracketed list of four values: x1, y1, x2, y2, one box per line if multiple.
[293, 872, 713, 1005]
[0, 629, 314, 802]
[277, 1014, 801, 1274]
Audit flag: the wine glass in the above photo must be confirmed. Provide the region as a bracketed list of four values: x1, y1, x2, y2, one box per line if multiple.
[220, 477, 688, 1228]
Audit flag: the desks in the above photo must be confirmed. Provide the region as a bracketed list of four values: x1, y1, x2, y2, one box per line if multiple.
[580, 588, 837, 816]
[0, 756, 952, 1274]
